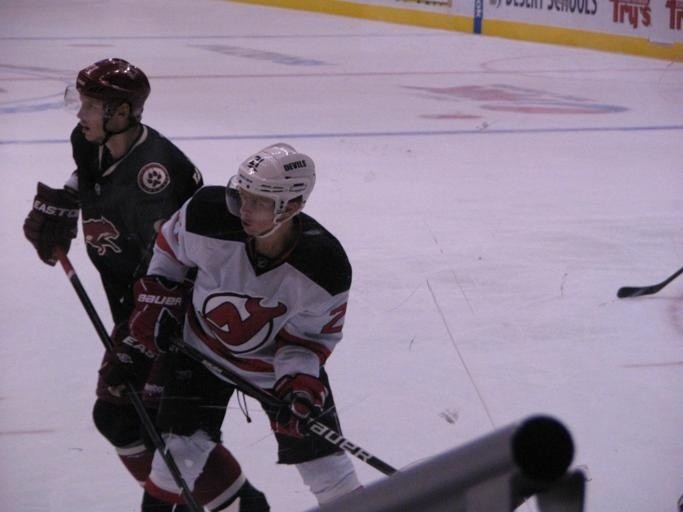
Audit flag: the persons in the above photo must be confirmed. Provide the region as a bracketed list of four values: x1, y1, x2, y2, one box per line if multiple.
[115, 138, 365, 512]
[19, 55, 273, 512]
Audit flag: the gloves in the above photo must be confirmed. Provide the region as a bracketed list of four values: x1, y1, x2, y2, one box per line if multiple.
[97, 337, 158, 396]
[23, 182, 79, 268]
[273, 372, 328, 438]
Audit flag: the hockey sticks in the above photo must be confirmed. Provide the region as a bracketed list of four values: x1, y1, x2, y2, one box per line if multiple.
[617, 268, 679, 297]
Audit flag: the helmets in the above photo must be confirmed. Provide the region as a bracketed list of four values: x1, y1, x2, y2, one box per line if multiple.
[77, 58, 150, 114]
[237, 143, 316, 202]
